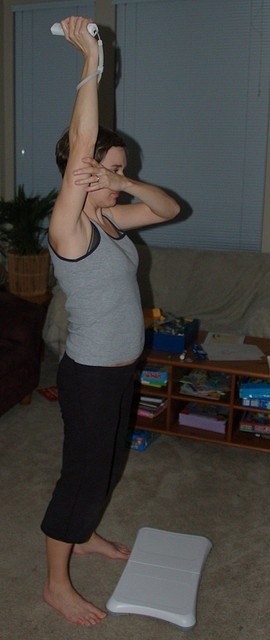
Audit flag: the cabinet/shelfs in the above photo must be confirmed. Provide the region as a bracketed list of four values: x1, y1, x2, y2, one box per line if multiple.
[133, 331, 269, 455]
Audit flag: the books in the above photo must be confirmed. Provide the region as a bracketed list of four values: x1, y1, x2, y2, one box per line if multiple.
[136, 395, 169, 419]
[242, 397, 270, 409]
[179, 405, 228, 434]
[178, 369, 231, 400]
[138, 365, 169, 388]
[240, 411, 270, 434]
[239, 383, 270, 399]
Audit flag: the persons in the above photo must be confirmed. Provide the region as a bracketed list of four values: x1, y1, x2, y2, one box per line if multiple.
[40, 17, 180, 626]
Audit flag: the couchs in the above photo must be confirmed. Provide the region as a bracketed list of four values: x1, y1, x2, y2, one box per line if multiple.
[0, 288, 43, 415]
[37, 243, 270, 404]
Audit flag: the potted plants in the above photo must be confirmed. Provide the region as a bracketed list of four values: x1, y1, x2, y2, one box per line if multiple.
[0, 182, 58, 305]
